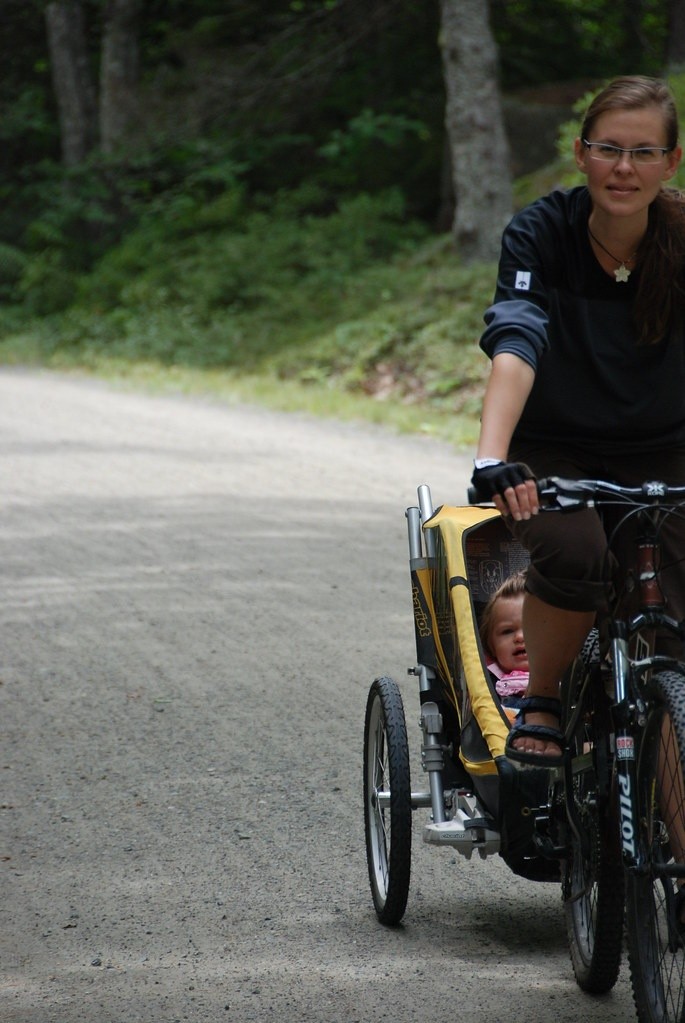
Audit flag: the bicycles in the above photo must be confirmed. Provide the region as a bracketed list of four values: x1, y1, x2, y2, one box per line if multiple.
[358, 463, 685, 1021]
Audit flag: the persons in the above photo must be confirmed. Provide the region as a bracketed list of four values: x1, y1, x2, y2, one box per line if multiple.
[482, 570, 594, 753]
[470, 77, 685, 947]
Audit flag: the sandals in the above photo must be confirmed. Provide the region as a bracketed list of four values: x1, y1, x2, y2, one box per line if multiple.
[504, 695, 567, 766]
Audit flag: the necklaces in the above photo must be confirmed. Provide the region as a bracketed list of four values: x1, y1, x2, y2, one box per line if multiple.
[588, 225, 637, 282]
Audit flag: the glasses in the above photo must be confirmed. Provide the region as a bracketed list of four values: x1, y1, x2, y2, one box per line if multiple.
[583, 139, 672, 165]
